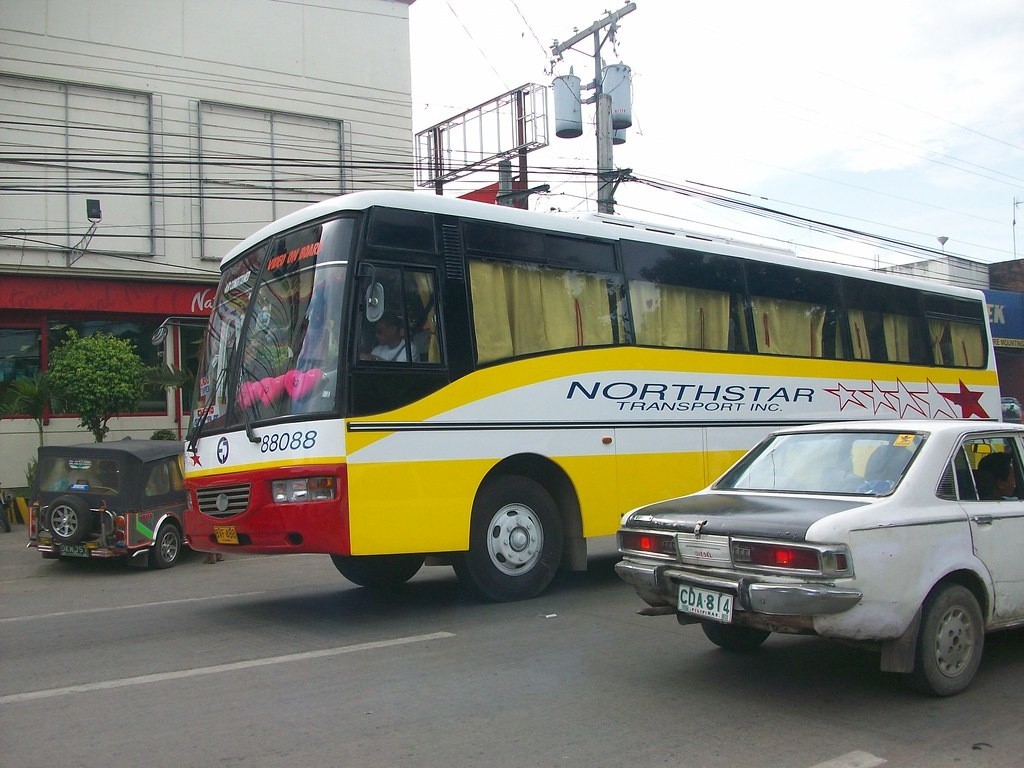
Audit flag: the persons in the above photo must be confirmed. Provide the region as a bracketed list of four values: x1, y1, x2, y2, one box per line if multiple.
[203, 552, 224, 564]
[977, 451, 1017, 500]
[361, 312, 420, 363]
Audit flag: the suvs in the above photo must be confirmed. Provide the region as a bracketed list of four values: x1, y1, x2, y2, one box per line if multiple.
[30, 436, 183, 569]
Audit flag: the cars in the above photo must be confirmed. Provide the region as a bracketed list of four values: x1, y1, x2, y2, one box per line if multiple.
[616, 417, 1023, 697]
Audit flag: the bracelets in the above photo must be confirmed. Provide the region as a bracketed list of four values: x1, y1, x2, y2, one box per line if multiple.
[373, 355, 378, 360]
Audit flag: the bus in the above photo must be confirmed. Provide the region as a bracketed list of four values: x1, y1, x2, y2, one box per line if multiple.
[182, 189, 1006, 602]
